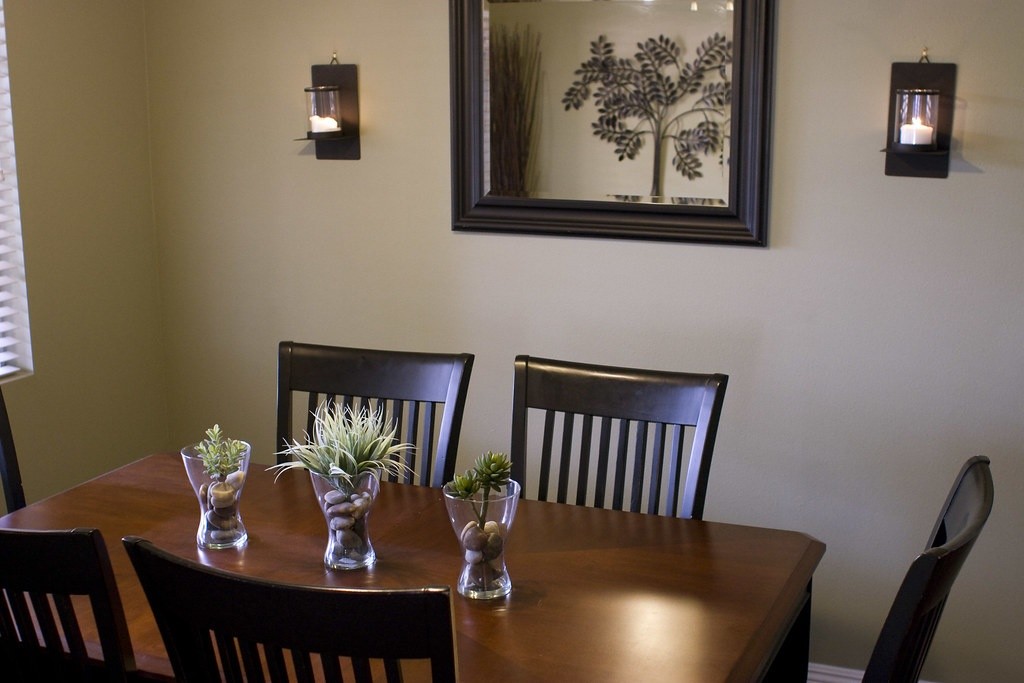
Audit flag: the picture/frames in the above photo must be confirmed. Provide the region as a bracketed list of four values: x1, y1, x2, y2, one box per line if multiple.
[448, 0, 775, 249]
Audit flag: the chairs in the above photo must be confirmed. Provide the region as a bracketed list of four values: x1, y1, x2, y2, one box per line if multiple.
[0, 529, 138, 683]
[276, 341, 475, 491]
[123, 536, 461, 683]
[809, 456, 994, 683]
[508, 355, 729, 523]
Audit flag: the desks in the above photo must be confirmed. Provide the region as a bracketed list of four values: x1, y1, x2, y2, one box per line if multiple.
[0, 451, 828, 683]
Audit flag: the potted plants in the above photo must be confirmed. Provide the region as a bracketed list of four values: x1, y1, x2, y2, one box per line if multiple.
[180, 425, 252, 551]
[264, 398, 420, 571]
[442, 450, 521, 600]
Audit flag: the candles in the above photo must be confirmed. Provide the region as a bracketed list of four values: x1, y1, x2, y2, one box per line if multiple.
[304, 85, 343, 138]
[889, 82, 943, 152]
